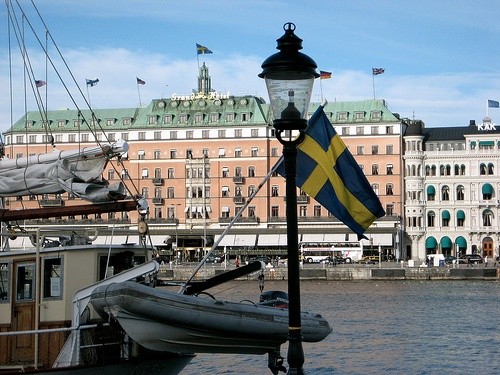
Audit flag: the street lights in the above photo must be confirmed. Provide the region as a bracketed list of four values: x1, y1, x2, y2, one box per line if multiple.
[256, 22, 320, 375]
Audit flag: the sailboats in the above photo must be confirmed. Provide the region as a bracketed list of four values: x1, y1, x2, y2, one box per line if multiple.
[0, 0, 200, 375]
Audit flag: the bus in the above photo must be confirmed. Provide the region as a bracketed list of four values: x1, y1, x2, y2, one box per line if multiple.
[298, 241, 363, 264]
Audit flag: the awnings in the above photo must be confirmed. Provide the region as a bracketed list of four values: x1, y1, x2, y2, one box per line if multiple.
[455, 236, 466, 247]
[442, 210, 450, 219]
[482, 184, 492, 194]
[457, 210, 465, 218]
[479, 141, 494, 145]
[427, 186, 434, 194]
[425, 236, 437, 248]
[440, 236, 452, 248]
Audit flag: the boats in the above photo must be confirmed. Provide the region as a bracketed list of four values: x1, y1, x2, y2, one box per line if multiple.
[90, 260, 334, 375]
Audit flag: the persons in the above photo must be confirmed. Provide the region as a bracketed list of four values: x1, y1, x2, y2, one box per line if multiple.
[485, 256, 488, 268]
[467, 256, 473, 267]
[493, 256, 497, 267]
[235, 258, 240, 268]
[426, 256, 434, 267]
[326, 255, 337, 267]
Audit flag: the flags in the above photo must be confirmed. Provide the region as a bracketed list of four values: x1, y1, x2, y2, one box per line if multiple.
[276, 109, 386, 241]
[137, 78, 145, 85]
[86, 79, 100, 87]
[373, 68, 384, 75]
[320, 71, 332, 80]
[488, 100, 499, 108]
[197, 44, 213, 55]
[35, 80, 45, 87]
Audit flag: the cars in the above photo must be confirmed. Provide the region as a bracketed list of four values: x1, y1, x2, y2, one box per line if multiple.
[445, 255, 457, 263]
[455, 254, 483, 264]
[202, 254, 224, 263]
[249, 256, 272, 264]
